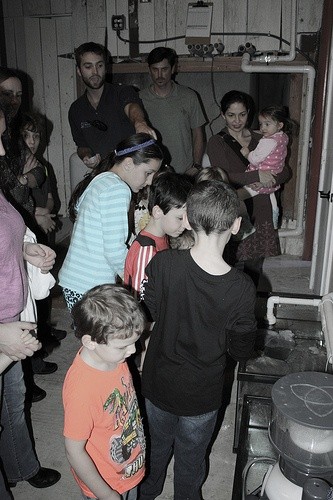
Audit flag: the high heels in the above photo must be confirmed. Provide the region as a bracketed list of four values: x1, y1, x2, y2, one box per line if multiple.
[8, 467, 61, 488]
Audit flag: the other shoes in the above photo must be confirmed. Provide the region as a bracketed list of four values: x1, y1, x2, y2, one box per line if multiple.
[32, 384, 46, 403]
[51, 327, 67, 341]
[33, 360, 58, 376]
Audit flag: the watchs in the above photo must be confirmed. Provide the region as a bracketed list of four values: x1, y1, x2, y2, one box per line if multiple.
[193, 163, 203, 171]
[21, 173, 28, 184]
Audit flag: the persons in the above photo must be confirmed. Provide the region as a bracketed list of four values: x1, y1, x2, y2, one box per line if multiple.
[0, 66, 65, 500]
[123, 179, 194, 381]
[169, 167, 232, 250]
[206, 89, 282, 261]
[61, 283, 147, 500]
[136, 47, 208, 178]
[235, 103, 291, 241]
[68, 42, 158, 169]
[136, 177, 258, 500]
[54, 131, 163, 315]
